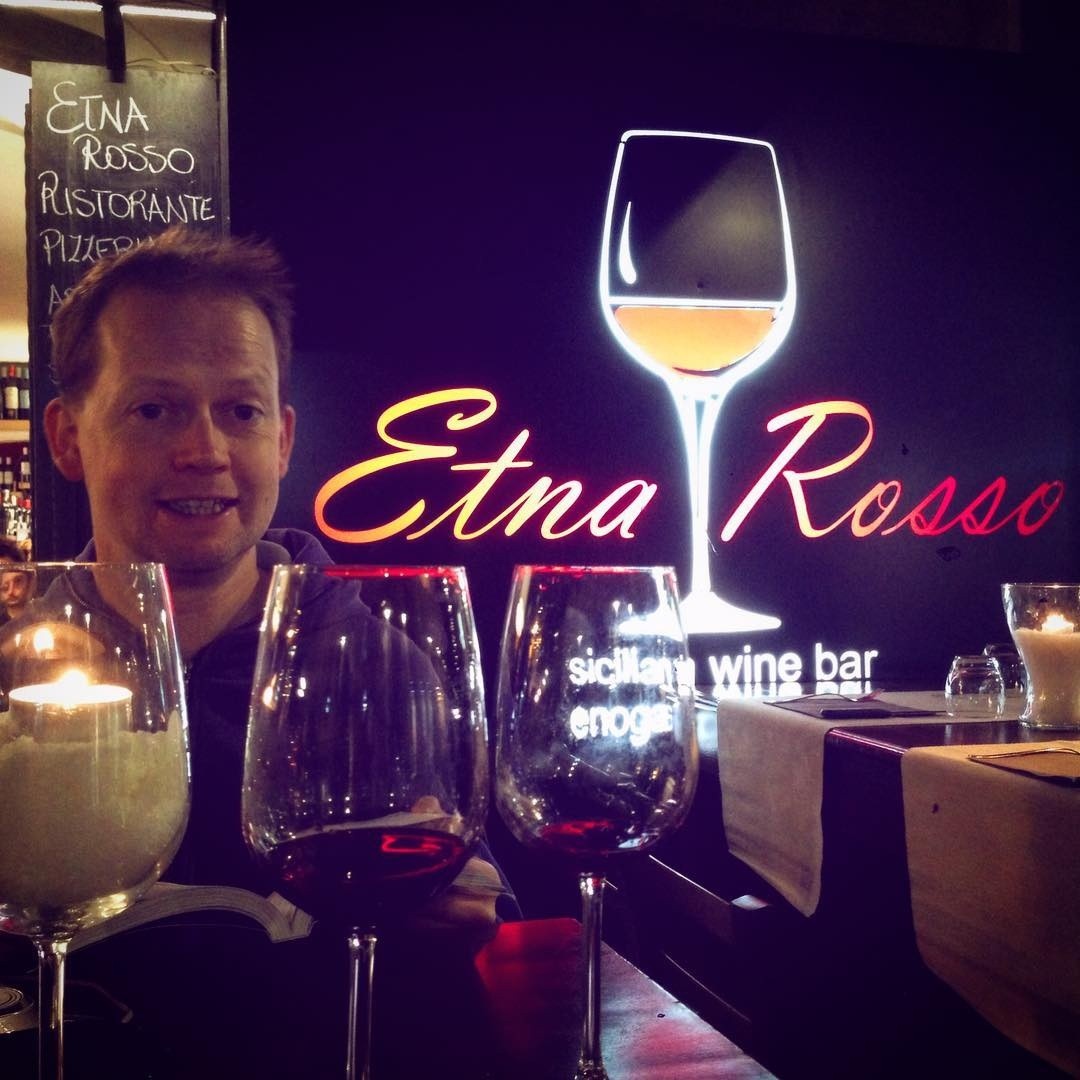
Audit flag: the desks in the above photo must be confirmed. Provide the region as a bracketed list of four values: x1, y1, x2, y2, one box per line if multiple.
[695, 680, 1080, 815]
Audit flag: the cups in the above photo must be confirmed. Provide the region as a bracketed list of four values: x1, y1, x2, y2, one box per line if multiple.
[944, 654, 1005, 721]
[999, 580, 1080, 732]
[981, 643, 1027, 699]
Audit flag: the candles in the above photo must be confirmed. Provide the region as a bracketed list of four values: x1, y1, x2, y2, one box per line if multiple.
[1014, 615, 1080, 725]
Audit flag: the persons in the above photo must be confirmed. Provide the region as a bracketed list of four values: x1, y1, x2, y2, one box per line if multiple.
[28, 227, 519, 1080]
[0, 542, 36, 619]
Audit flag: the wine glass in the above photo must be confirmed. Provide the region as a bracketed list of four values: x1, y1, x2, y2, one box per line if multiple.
[491, 563, 704, 1080]
[238, 561, 486, 1080]
[2, 559, 193, 1080]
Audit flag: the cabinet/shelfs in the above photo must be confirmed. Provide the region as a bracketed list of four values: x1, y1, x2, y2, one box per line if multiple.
[0, 419, 30, 547]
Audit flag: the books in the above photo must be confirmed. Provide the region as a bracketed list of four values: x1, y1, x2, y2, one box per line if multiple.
[64, 858, 506, 944]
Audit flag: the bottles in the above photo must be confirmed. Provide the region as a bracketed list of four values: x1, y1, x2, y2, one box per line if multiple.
[0, 364, 30, 421]
[0, 446, 32, 540]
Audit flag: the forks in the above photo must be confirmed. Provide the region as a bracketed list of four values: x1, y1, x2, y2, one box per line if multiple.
[763, 686, 887, 704]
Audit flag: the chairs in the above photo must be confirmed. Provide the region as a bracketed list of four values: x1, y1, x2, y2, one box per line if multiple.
[633, 850, 779, 1069]
